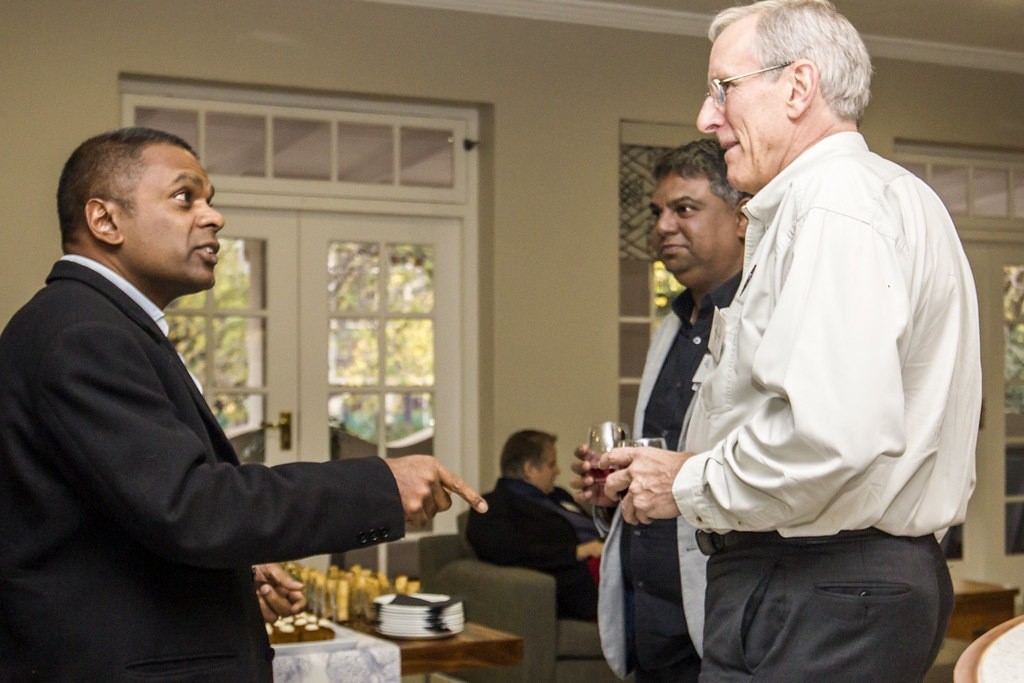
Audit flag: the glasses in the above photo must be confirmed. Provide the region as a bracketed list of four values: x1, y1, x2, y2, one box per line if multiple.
[699, 58, 796, 107]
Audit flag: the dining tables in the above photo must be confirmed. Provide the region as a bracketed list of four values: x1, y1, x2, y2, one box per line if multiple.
[263, 593, 525, 683]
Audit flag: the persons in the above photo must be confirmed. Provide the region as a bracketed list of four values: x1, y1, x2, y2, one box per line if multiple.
[570, 137, 755, 683]
[468, 427, 606, 622]
[597, 0, 984, 683]
[0, 125, 489, 683]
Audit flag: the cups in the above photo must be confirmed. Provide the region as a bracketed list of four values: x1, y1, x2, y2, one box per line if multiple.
[618, 438, 670, 506]
[586, 421, 630, 484]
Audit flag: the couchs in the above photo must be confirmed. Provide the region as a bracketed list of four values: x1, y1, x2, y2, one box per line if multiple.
[414, 507, 621, 683]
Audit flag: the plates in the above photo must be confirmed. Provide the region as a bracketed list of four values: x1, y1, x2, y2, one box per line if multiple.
[371, 592, 466, 640]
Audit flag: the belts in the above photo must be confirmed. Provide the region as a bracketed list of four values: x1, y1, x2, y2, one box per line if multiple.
[692, 521, 898, 557]
